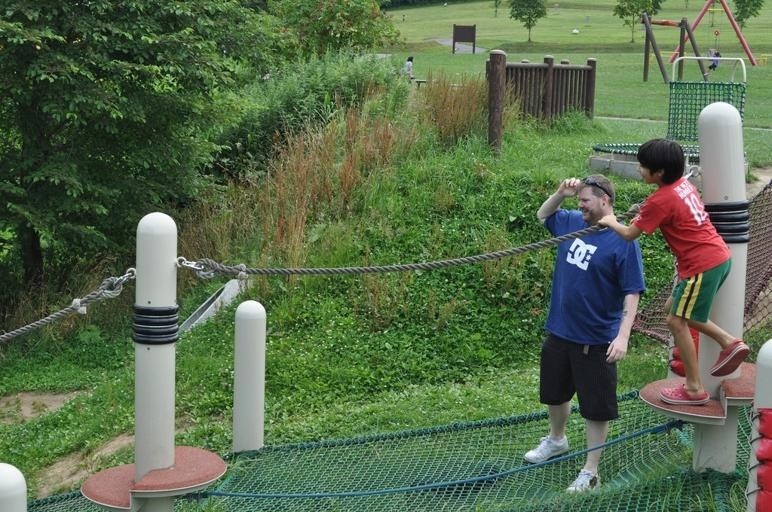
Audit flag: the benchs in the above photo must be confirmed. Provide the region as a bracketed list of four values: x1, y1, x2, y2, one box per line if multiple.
[414, 78, 439, 89]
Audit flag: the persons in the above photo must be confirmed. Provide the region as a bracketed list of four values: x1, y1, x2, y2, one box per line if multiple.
[705, 52, 721, 77]
[403, 55, 413, 80]
[599, 137, 751, 408]
[521, 173, 646, 496]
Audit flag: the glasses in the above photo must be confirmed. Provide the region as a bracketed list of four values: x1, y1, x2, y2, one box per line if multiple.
[580, 177, 613, 199]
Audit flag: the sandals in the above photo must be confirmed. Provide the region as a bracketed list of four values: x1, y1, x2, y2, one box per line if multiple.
[658, 383, 712, 407]
[708, 337, 750, 377]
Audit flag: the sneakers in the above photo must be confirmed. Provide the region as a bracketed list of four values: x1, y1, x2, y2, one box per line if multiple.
[524, 433, 570, 465]
[566, 468, 602, 496]
